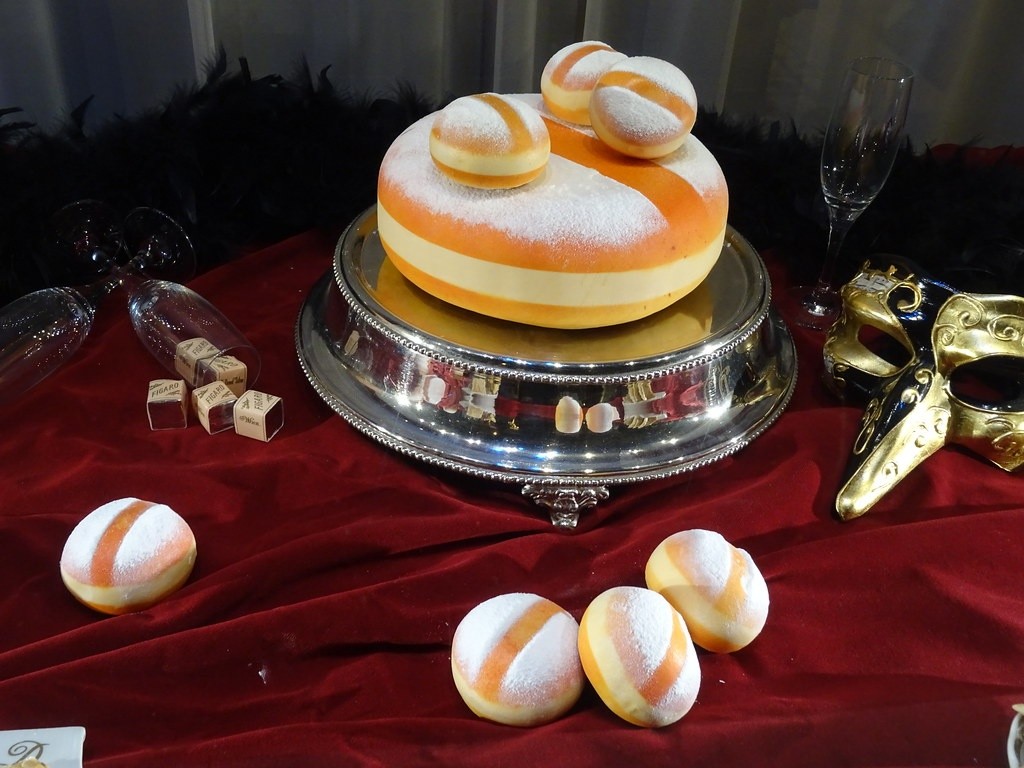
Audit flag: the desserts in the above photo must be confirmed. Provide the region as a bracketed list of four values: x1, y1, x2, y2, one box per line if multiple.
[450, 529, 769, 728]
[59, 496, 197, 615]
[377, 39, 729, 331]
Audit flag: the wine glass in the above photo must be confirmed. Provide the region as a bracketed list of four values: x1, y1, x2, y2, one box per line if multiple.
[35, 197, 262, 408]
[0, 206, 196, 424]
[784, 58, 916, 327]
